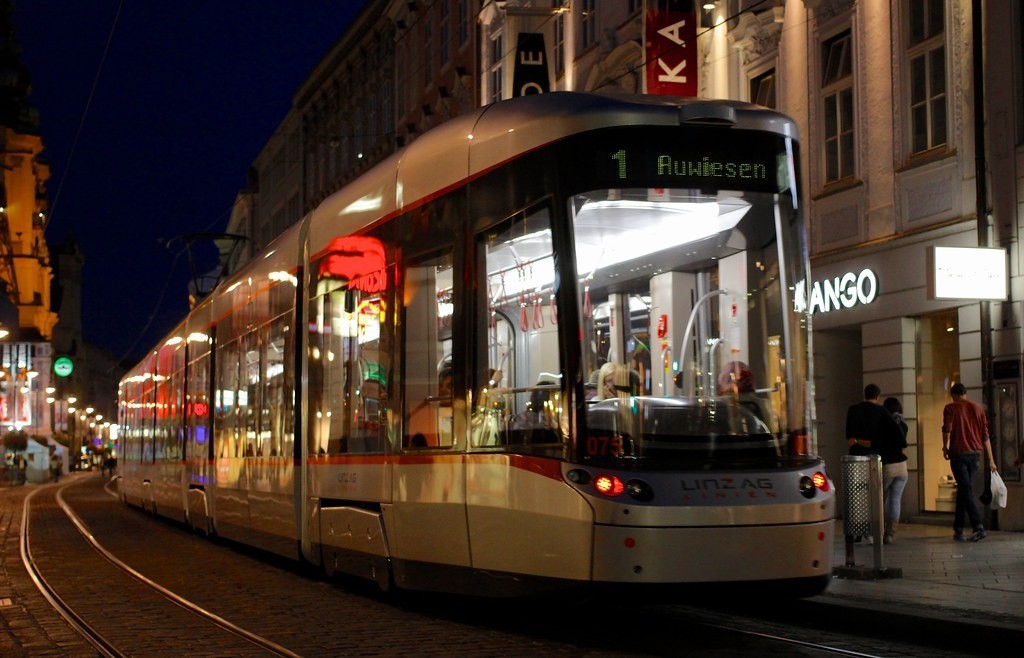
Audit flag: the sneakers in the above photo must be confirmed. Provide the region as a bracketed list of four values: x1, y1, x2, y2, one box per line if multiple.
[953, 532, 963, 540]
[967, 527, 986, 542]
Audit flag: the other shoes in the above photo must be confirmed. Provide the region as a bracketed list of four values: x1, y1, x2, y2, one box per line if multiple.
[866, 536, 873, 545]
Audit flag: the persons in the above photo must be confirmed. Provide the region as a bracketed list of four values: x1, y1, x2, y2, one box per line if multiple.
[585, 362, 621, 408]
[502, 380, 555, 430]
[359, 378, 389, 423]
[942, 383, 997, 542]
[51, 455, 60, 483]
[845, 383, 909, 546]
[718, 361, 764, 423]
[100, 454, 116, 476]
[436, 351, 504, 408]
[17, 455, 28, 485]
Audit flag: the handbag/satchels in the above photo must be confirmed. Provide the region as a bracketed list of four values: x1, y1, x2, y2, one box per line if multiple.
[990, 469, 1007, 509]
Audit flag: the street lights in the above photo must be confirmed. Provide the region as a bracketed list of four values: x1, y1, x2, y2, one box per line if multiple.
[34, 383, 54, 437]
[79, 406, 94, 472]
[60, 395, 77, 433]
[9, 366, 40, 486]
[90, 413, 104, 465]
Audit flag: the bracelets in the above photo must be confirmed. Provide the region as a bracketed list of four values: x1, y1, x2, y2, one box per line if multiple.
[854, 439, 861, 444]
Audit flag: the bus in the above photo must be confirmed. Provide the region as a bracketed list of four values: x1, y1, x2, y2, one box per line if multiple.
[111, 88, 840, 609]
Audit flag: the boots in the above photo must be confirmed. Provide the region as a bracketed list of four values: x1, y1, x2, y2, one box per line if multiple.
[883, 516, 899, 544]
